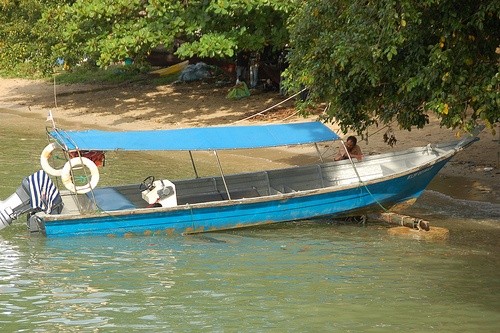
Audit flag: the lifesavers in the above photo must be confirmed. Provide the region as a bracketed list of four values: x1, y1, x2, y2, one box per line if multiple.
[61, 157, 101, 195]
[39, 142, 63, 177]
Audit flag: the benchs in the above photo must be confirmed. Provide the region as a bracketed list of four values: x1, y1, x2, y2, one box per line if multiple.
[86, 188, 137, 211]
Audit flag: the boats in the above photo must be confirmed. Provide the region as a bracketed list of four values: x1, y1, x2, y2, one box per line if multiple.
[27, 122, 481, 241]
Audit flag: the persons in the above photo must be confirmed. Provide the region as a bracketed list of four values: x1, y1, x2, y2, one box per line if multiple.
[334, 136, 362, 162]
[236, 49, 248, 85]
[279, 46, 289, 96]
[248, 51, 260, 89]
